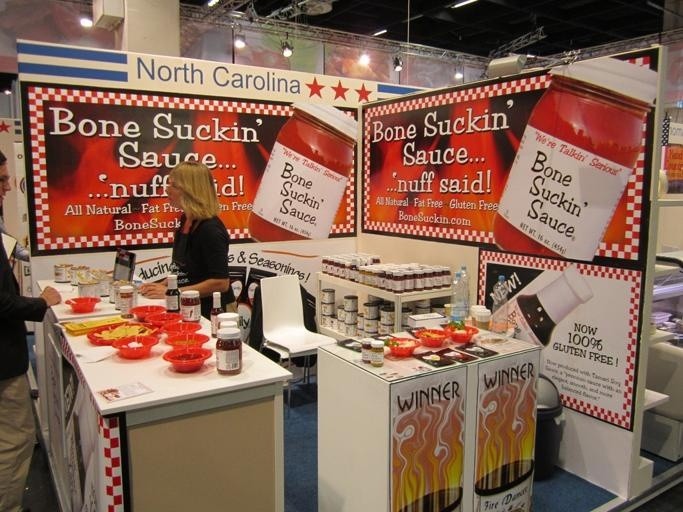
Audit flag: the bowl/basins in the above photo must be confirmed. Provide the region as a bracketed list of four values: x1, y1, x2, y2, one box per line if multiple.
[112, 305, 214, 374]
[65, 298, 101, 311]
[386, 324, 479, 356]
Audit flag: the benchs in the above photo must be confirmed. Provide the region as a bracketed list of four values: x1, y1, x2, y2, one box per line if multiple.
[640, 343, 682, 465]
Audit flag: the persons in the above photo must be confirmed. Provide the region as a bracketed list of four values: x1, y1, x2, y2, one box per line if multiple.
[0, 209, 30, 262]
[135, 161, 235, 319]
[0, 150, 61, 511]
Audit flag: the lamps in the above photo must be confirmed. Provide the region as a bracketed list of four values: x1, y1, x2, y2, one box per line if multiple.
[68, 0, 466, 81]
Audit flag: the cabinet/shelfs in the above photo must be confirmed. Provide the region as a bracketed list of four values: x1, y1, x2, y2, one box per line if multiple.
[648, 249, 683, 349]
[312, 268, 457, 340]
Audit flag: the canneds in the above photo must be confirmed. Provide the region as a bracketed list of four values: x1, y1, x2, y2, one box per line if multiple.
[54, 263, 134, 319]
[215, 311, 243, 376]
[492, 57, 657, 263]
[180, 290, 202, 324]
[321, 254, 451, 368]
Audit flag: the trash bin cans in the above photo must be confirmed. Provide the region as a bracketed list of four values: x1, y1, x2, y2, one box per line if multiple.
[533, 373, 562, 482]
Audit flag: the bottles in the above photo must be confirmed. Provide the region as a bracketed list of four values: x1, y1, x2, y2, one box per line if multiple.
[166, 275, 180, 313]
[210, 290, 242, 375]
[361, 338, 385, 369]
[452, 266, 472, 329]
[497, 268, 594, 353]
[119, 286, 133, 318]
[322, 249, 452, 294]
[471, 302, 491, 329]
[492, 263, 510, 332]
[181, 289, 201, 323]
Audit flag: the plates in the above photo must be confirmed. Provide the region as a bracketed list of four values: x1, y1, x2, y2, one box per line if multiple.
[87, 320, 164, 347]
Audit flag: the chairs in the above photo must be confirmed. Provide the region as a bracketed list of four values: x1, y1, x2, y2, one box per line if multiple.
[253, 273, 333, 412]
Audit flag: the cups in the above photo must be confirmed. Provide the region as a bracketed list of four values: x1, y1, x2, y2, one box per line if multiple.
[400, 458, 534, 511]
[444, 303, 452, 314]
